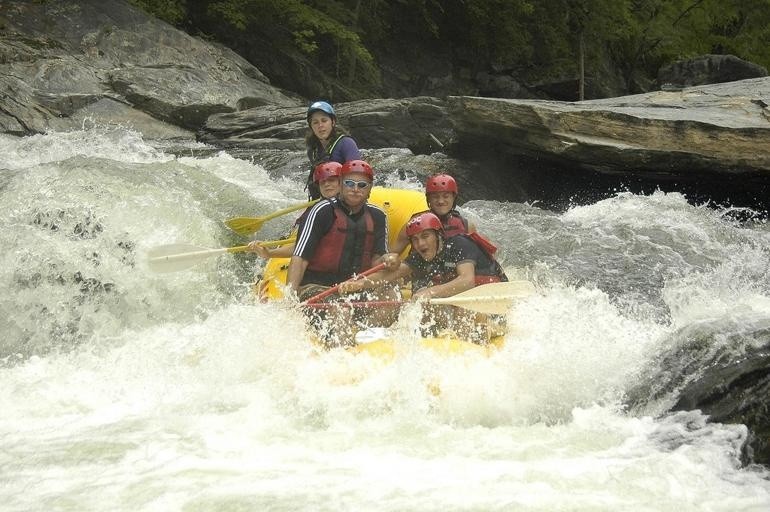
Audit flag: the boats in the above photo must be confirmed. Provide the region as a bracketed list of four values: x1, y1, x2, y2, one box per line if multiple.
[255, 186, 506, 357]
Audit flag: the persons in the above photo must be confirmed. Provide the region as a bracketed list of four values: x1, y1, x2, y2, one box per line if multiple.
[338, 214, 509, 344]
[282, 159, 402, 346]
[304, 101, 361, 199]
[390, 173, 476, 254]
[247, 161, 344, 259]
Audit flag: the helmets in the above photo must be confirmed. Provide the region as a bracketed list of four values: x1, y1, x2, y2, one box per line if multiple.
[406, 212, 445, 237]
[342, 159, 375, 178]
[423, 173, 459, 195]
[312, 161, 343, 184]
[307, 100, 337, 121]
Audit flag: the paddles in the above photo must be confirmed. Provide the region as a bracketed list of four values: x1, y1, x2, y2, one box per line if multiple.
[146, 237, 296, 273]
[224, 199, 320, 238]
[300, 280, 535, 314]
